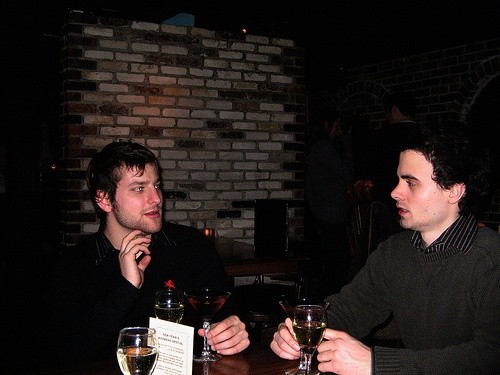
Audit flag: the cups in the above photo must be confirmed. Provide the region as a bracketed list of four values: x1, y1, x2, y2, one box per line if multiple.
[116, 327, 158, 375]
[152, 288, 183, 324]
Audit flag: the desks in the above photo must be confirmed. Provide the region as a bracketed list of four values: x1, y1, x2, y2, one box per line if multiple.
[215, 236, 306, 288]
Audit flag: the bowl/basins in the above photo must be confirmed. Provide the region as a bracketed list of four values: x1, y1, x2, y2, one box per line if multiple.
[248, 313, 271, 330]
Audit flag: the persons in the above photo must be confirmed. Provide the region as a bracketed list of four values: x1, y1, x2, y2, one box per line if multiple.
[301, 93, 427, 303]
[269, 122, 500, 375]
[44, 141, 251, 366]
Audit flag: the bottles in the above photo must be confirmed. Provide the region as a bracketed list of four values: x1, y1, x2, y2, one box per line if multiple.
[295, 268, 309, 301]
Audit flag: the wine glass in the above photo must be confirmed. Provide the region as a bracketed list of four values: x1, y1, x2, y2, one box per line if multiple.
[183, 288, 232, 362]
[292, 305, 326, 375]
